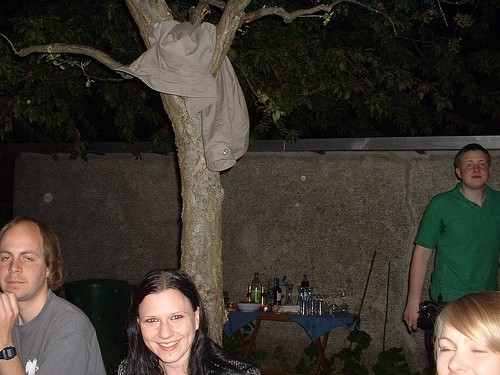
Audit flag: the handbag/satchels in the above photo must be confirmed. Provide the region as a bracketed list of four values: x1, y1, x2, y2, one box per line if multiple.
[417, 298, 444, 330]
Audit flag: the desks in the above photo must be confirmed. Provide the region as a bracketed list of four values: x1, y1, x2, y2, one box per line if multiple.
[224, 311, 354, 375]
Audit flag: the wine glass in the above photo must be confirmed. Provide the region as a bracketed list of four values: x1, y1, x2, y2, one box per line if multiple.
[339, 292, 350, 314]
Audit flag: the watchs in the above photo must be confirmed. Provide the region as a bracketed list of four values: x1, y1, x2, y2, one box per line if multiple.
[0, 345, 17, 361]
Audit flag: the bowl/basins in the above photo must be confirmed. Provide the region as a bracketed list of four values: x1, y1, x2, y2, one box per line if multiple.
[281, 305, 299, 312]
[237, 303, 260, 312]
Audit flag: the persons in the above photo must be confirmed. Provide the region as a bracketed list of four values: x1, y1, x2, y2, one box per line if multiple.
[118, 268, 263, 375]
[402, 144, 500, 334]
[0, 216, 108, 375]
[432, 292, 500, 375]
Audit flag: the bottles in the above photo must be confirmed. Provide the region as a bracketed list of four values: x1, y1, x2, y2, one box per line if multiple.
[251, 271, 262, 304]
[283, 283, 295, 305]
[306, 298, 313, 316]
[269, 277, 283, 306]
[300, 274, 309, 304]
[329, 293, 339, 316]
[319, 298, 324, 315]
[315, 298, 319, 314]
